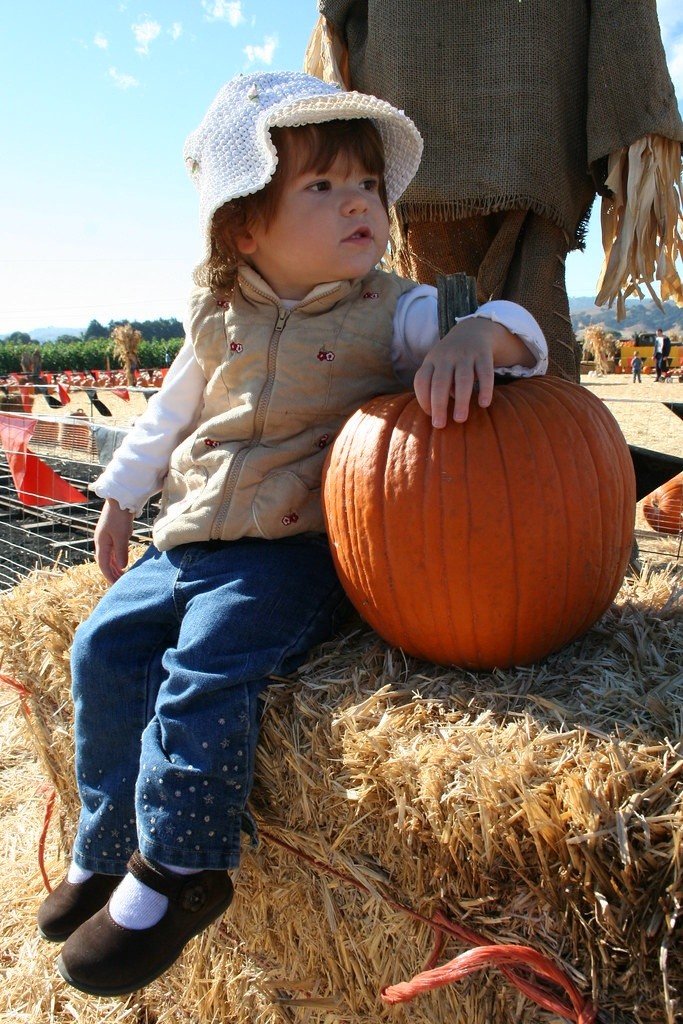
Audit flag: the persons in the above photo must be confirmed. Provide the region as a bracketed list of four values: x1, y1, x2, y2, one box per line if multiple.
[651, 328, 671, 382]
[165, 350, 172, 367]
[38, 69, 550, 997]
[631, 350, 643, 383]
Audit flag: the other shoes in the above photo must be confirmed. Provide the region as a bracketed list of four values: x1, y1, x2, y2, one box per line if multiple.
[57, 849, 234, 997]
[38, 852, 124, 943]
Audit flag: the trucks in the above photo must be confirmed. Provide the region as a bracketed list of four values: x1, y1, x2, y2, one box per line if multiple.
[613, 332, 683, 364]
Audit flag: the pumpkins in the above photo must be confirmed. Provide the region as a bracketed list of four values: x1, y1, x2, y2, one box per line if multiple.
[643, 366, 651, 373]
[62, 374, 163, 387]
[320, 271, 637, 671]
[643, 478, 683, 533]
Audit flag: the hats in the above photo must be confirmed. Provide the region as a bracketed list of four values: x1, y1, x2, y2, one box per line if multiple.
[183, 71, 425, 290]
